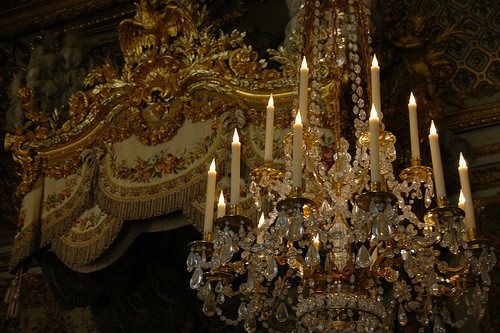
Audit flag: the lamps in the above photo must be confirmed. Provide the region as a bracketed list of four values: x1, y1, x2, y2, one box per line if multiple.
[186, 0, 498, 333]
